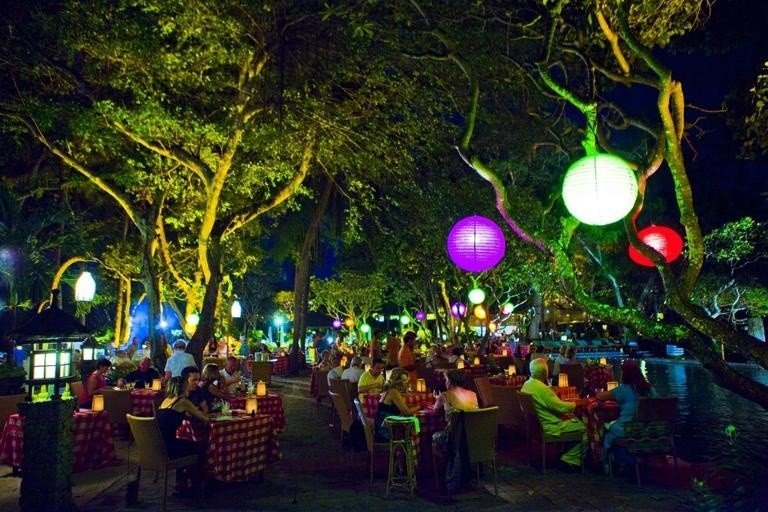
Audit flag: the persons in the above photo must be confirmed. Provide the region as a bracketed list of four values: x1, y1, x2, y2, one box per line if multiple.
[74, 329, 658, 500]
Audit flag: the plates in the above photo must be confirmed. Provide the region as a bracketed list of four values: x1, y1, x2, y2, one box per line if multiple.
[255, 353, 269, 362]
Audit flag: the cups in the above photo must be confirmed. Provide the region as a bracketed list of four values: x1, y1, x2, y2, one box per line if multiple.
[433, 389, 441, 399]
[145, 384, 149, 390]
[547, 378, 552, 387]
[247, 385, 254, 395]
[222, 402, 230, 415]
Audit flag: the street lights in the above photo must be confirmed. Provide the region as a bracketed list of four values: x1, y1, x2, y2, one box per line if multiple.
[275, 318, 280, 346]
[5, 287, 93, 511]
[223, 293, 241, 358]
[76, 263, 96, 328]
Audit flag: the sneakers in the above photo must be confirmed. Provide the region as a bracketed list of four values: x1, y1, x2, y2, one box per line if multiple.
[560, 459, 582, 473]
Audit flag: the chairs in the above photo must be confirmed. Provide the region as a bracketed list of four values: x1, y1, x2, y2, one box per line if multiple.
[0, 335, 690, 511]
